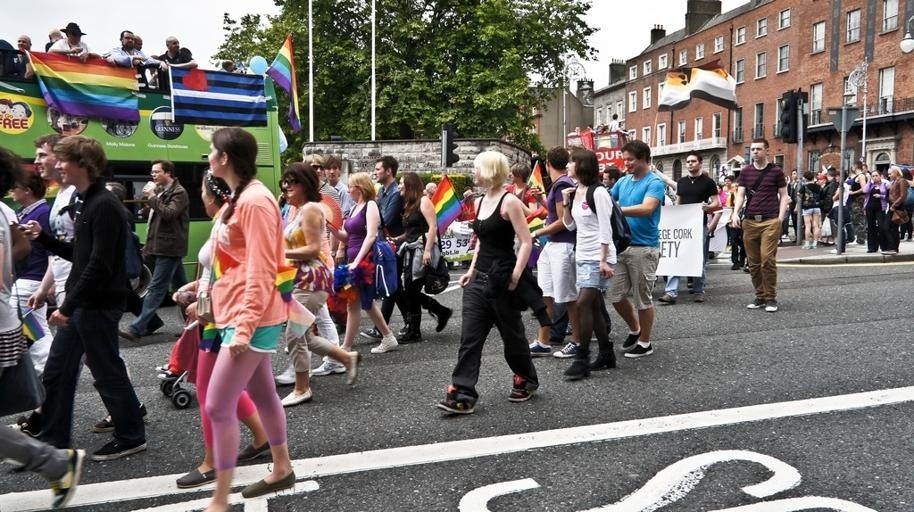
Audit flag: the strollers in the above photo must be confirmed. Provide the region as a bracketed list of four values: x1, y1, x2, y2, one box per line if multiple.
[160, 290, 199, 409]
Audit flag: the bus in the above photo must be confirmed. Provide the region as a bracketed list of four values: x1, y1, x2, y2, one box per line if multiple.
[0, 49, 285, 312]
[566, 128, 629, 181]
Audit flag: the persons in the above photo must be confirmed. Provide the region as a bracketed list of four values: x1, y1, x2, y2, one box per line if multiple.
[44, 29, 63, 52]
[438, 150, 539, 413]
[608, 114, 625, 134]
[454, 139, 664, 378]
[587, 123, 607, 134]
[101, 30, 241, 74]
[1, 125, 455, 512]
[47, 22, 88, 56]
[778, 160, 913, 255]
[17, 34, 32, 51]
[650, 152, 749, 302]
[731, 139, 786, 311]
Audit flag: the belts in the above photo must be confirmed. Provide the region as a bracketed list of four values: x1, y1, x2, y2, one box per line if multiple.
[746, 215, 769, 223]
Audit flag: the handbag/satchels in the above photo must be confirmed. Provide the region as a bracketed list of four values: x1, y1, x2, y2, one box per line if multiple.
[195, 290, 214, 325]
[424, 256, 450, 294]
[891, 208, 910, 223]
[802, 186, 817, 208]
[119, 221, 144, 279]
[0, 352, 48, 417]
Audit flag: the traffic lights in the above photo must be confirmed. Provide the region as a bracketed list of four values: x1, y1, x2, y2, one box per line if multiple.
[440, 124, 459, 168]
[779, 90, 796, 144]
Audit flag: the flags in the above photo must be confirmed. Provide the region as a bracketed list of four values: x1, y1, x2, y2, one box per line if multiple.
[656, 58, 742, 112]
[24, 49, 140, 122]
[265, 35, 302, 133]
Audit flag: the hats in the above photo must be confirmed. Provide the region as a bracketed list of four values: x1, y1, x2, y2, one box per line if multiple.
[60, 22, 86, 36]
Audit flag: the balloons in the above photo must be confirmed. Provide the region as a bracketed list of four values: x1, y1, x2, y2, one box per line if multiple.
[249, 55, 268, 75]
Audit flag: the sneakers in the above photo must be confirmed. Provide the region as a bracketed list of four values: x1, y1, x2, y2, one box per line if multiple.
[747, 298, 766, 309]
[792, 236, 834, 249]
[508, 390, 533, 402]
[242, 470, 295, 499]
[360, 298, 452, 355]
[881, 250, 897, 255]
[765, 301, 778, 312]
[437, 400, 475, 413]
[237, 440, 271, 461]
[4, 418, 51, 445]
[47, 449, 86, 509]
[694, 294, 704, 302]
[658, 293, 676, 302]
[91, 435, 147, 462]
[528, 323, 654, 379]
[273, 349, 361, 407]
[94, 402, 148, 433]
[118, 329, 138, 344]
[155, 366, 183, 381]
[177, 468, 218, 488]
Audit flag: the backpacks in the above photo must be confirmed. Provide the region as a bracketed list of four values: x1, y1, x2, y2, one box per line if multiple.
[569, 183, 632, 254]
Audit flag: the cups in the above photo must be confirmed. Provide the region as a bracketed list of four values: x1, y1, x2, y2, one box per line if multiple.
[141, 180, 158, 197]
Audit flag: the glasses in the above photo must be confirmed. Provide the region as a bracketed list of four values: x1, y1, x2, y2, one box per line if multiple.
[283, 178, 299, 186]
[312, 165, 325, 171]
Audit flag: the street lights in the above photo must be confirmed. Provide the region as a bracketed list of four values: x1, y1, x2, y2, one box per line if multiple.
[842, 56, 868, 170]
[899, 15, 913, 55]
[562, 52, 592, 151]
